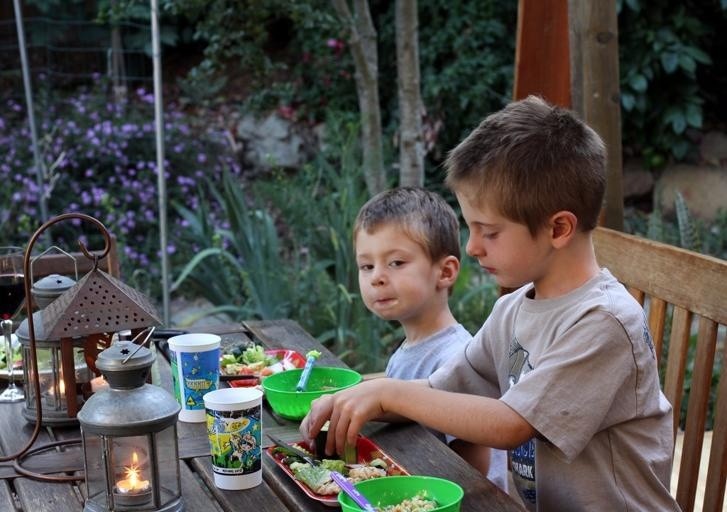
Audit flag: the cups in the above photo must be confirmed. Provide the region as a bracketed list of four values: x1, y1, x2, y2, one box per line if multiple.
[164, 330, 223, 427]
[201, 386, 265, 492]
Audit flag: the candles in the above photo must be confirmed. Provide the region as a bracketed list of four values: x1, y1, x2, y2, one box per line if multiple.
[116, 479, 150, 494]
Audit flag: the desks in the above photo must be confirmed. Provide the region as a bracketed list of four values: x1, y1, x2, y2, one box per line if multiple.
[1, 318, 528, 511]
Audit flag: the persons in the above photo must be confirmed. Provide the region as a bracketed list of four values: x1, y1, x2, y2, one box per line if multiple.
[296, 97, 682, 510]
[352, 186, 508, 495]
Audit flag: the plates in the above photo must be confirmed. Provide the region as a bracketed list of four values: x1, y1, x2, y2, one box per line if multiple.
[218, 348, 309, 389]
[265, 432, 414, 507]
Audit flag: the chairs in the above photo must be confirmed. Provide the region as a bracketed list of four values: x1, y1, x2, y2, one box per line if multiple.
[592, 224, 727, 512]
[1, 233, 120, 335]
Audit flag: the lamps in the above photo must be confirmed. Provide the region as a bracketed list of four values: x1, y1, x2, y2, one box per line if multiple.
[1, 211, 164, 484]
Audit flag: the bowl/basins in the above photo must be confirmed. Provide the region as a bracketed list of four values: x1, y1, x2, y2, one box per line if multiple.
[262, 365, 365, 424]
[336, 473, 467, 512]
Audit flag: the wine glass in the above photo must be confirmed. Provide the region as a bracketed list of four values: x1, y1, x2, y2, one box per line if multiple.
[0, 245, 28, 403]
